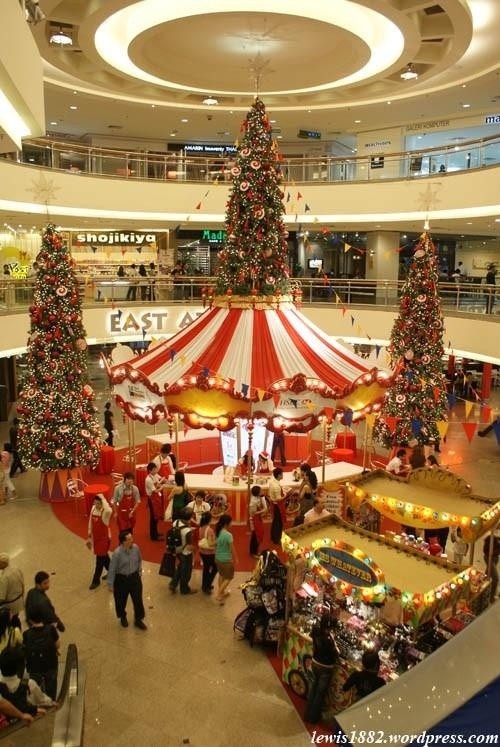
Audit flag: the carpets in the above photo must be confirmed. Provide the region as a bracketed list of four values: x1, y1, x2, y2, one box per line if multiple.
[51, 439, 390, 572]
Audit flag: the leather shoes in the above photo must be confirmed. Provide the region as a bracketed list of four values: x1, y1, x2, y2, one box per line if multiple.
[121, 616, 128, 626]
[135, 620, 147, 630]
[90, 580, 100, 589]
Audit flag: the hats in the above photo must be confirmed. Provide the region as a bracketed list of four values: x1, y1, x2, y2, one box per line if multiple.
[260, 450, 268, 459]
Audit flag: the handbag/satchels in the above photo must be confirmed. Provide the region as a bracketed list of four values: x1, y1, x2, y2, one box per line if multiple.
[198, 538, 215, 550]
[159, 552, 176, 577]
[234, 550, 287, 644]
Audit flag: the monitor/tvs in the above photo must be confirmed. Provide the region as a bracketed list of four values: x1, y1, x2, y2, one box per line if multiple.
[308, 259, 323, 269]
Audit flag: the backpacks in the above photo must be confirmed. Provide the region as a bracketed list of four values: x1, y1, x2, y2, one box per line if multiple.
[166, 521, 189, 550]
[0, 678, 38, 724]
[25, 626, 56, 681]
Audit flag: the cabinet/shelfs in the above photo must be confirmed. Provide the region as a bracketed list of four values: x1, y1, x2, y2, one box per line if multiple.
[143, 430, 309, 467]
[161, 461, 370, 527]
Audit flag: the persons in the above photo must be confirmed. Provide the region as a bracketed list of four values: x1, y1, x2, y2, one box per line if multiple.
[117, 261, 189, 302]
[28, 262, 41, 286]
[104, 402, 115, 446]
[3, 264, 10, 274]
[484, 263, 497, 314]
[440, 262, 467, 282]
[9, 417, 28, 479]
[0, 434, 500, 747]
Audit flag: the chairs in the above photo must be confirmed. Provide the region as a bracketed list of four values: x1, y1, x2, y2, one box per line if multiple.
[315, 423, 457, 478]
[67, 440, 188, 523]
